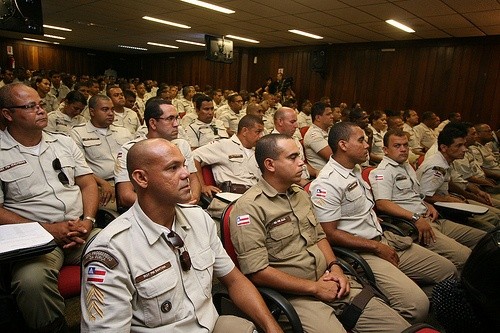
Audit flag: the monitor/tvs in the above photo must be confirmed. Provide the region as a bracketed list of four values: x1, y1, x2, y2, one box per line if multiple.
[204, 34, 234, 64]
[0, 0, 44, 35]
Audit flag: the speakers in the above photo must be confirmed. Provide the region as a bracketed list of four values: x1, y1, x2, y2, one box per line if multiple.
[309, 48, 331, 73]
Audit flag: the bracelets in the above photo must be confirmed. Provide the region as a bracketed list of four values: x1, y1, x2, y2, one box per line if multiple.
[328, 260, 342, 269]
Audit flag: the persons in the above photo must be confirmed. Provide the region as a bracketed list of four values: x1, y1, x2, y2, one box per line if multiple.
[320, 97, 440, 167]
[0, 83, 101, 333]
[229, 134, 413, 333]
[0, 69, 314, 153]
[271, 107, 321, 189]
[81, 138, 287, 333]
[193, 114, 264, 198]
[309, 123, 458, 323]
[368, 130, 487, 270]
[416, 111, 500, 241]
[303, 102, 334, 171]
[114, 100, 201, 205]
[70, 93, 132, 214]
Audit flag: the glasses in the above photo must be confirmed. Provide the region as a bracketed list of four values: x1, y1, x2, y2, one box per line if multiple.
[154, 116, 178, 121]
[52, 158, 69, 185]
[6, 102, 47, 110]
[167, 230, 191, 272]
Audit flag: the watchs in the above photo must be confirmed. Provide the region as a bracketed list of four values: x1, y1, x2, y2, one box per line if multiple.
[83, 217, 97, 227]
[412, 213, 420, 222]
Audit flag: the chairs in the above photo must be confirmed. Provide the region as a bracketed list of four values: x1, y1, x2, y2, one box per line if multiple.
[58, 210, 118, 295]
[202, 164, 490, 333]
